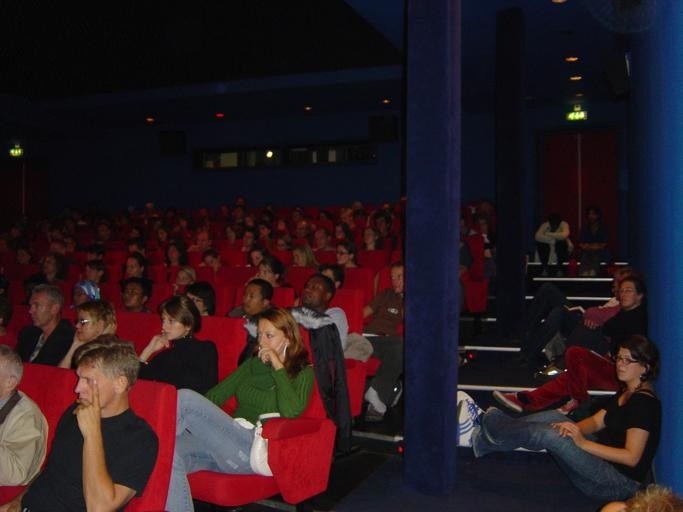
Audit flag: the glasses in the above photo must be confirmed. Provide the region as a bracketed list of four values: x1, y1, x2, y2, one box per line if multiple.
[336, 249, 353, 256]
[616, 287, 639, 296]
[74, 319, 90, 326]
[192, 297, 204, 304]
[391, 274, 404, 280]
[612, 354, 638, 364]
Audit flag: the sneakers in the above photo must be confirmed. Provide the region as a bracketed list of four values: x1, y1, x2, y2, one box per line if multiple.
[456, 390, 484, 423]
[389, 382, 402, 406]
[556, 399, 578, 415]
[534, 365, 563, 378]
[493, 390, 523, 412]
[455, 399, 475, 448]
[365, 402, 383, 421]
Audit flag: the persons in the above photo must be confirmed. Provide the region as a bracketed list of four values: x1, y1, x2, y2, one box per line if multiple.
[8, 334, 159, 512]
[455, 335, 661, 503]
[575, 207, 608, 275]
[534, 212, 574, 276]
[597, 483, 683, 512]
[491, 269, 683, 413]
[1, 195, 495, 415]
[0, 344, 50, 512]
[163, 307, 316, 512]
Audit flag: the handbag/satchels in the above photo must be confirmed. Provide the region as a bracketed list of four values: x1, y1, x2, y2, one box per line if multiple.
[249, 427, 274, 476]
